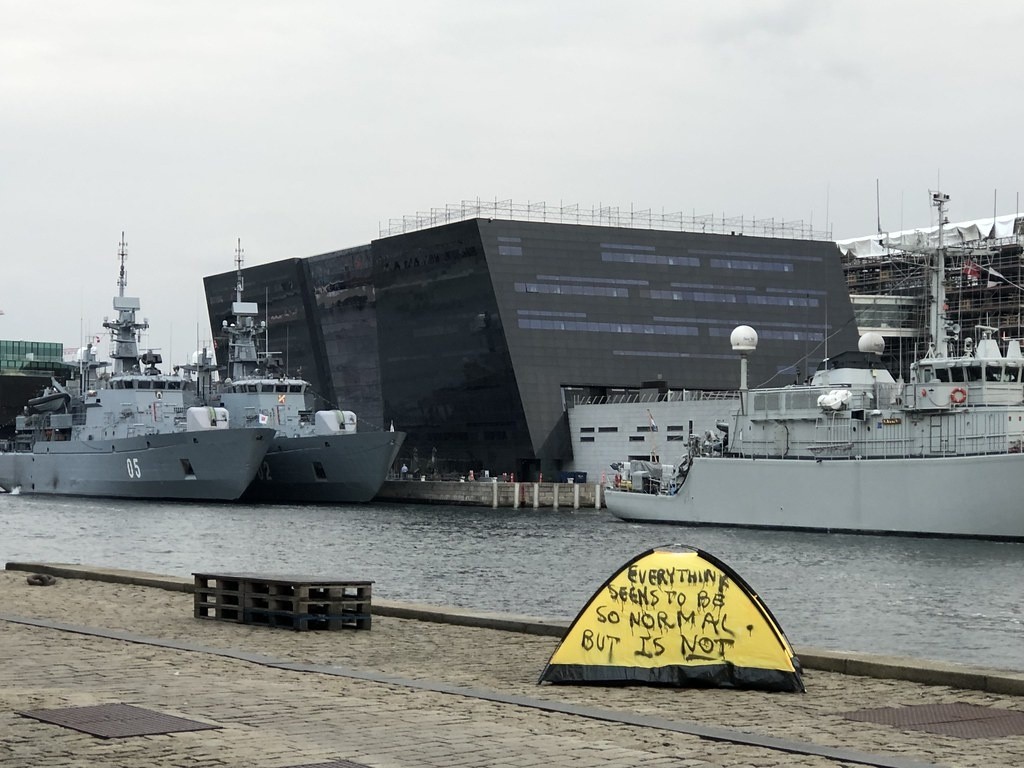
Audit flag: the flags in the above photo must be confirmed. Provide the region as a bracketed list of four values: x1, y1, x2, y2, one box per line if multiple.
[650, 413, 656, 430]
[259, 414, 268, 424]
[963, 260, 977, 276]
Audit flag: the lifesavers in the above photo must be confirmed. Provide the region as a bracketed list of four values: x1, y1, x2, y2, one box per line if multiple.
[614, 474, 622, 487]
[950, 387, 966, 404]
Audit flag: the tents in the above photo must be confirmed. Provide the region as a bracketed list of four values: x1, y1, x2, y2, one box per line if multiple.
[536, 544, 807, 693]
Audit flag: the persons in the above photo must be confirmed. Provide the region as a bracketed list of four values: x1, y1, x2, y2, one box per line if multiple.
[401, 463, 408, 480]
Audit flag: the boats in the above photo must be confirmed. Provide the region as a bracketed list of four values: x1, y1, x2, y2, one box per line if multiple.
[0, 231, 278, 504]
[601, 177, 1024, 547]
[180, 237, 407, 506]
